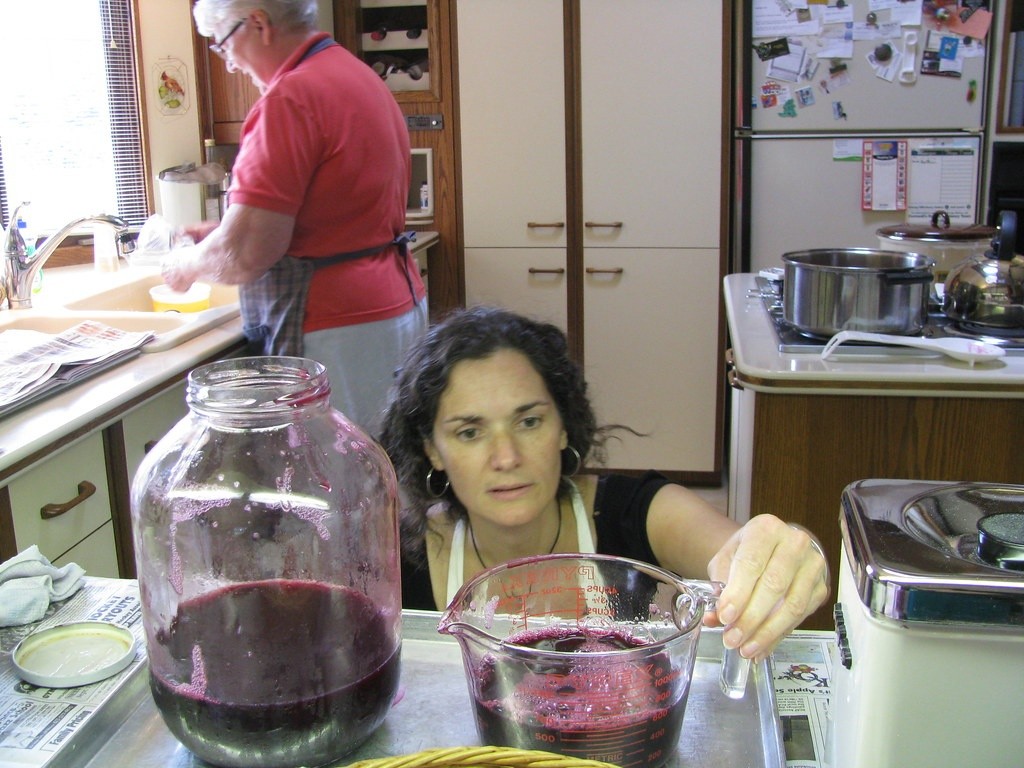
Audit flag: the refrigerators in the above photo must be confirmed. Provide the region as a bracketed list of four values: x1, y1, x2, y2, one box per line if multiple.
[734, 0, 994, 275]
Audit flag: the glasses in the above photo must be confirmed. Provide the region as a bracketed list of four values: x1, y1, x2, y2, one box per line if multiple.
[208, 10, 273, 61]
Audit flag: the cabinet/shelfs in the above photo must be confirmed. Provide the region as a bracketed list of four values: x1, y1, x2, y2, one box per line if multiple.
[725, 352, 1024, 631]
[443, 0, 731, 487]
[190, 0, 454, 236]
[0, 348, 248, 575]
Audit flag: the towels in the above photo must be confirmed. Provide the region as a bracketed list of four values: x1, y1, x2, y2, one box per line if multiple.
[0, 543, 88, 628]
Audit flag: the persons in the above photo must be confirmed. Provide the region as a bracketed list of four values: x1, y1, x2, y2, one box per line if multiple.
[162, 0, 427, 546]
[373, 305, 832, 665]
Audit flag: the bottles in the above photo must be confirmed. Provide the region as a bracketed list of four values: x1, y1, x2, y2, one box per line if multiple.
[203, 139, 222, 224]
[132, 357, 405, 768]
[420, 181, 428, 209]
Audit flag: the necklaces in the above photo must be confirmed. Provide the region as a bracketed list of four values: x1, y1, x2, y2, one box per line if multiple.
[468, 493, 562, 602]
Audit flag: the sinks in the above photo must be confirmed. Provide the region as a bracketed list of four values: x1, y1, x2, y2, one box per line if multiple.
[59, 268, 246, 321]
[0, 310, 225, 351]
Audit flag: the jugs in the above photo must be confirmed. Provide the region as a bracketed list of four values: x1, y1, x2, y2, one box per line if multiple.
[437, 552, 752, 768]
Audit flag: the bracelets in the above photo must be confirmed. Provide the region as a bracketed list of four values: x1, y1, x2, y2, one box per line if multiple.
[785, 520, 830, 585]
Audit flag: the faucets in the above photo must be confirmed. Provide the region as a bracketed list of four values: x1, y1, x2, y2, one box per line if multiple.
[0, 198, 137, 311]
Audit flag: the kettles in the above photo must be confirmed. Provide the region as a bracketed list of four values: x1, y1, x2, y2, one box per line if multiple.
[940, 211, 1024, 328]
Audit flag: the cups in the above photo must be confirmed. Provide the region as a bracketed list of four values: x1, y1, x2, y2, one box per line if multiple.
[148, 281, 211, 314]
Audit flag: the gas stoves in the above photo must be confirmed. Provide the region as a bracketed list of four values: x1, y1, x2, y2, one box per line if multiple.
[755, 276, 1024, 353]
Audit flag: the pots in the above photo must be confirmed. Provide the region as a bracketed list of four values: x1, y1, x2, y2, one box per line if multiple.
[874, 208, 996, 278]
[781, 247, 938, 343]
